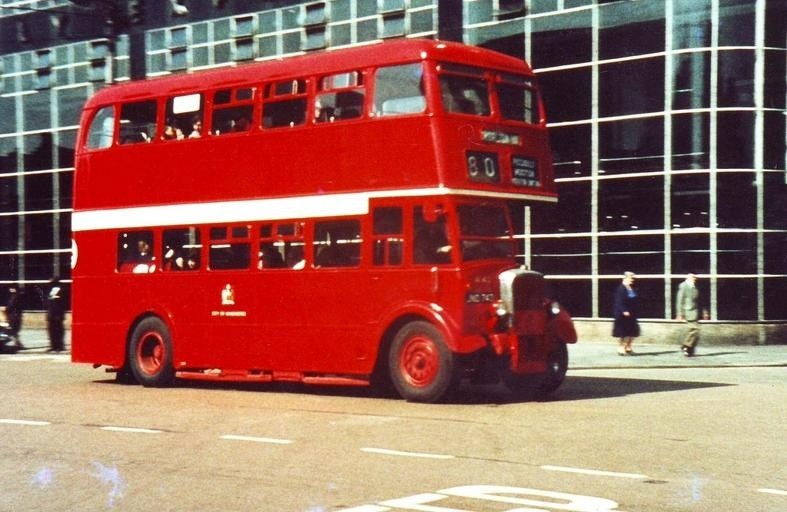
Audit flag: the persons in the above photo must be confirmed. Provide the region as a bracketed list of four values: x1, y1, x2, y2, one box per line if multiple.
[189, 117, 203, 139]
[613, 271, 642, 357]
[44, 273, 68, 352]
[164, 115, 185, 141]
[0, 282, 22, 348]
[676, 271, 710, 356]
[121, 237, 156, 273]
[184, 252, 200, 269]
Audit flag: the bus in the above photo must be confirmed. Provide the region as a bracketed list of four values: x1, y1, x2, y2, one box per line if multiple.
[69, 39, 578, 404]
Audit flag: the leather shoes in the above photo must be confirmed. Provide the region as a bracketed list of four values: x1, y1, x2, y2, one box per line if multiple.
[617, 343, 693, 357]
